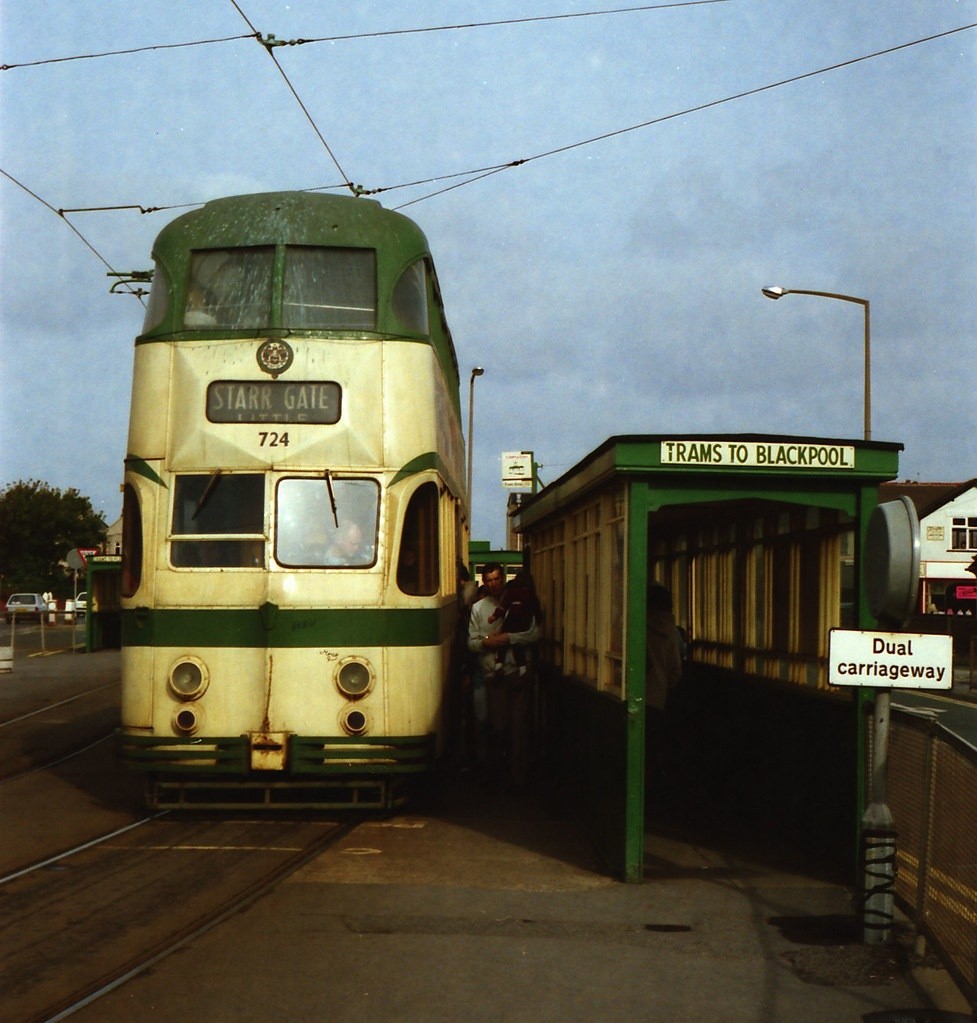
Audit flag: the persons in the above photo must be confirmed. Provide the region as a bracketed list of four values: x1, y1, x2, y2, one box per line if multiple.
[306, 522, 370, 564]
[466, 562, 543, 795]
[645, 586, 682, 812]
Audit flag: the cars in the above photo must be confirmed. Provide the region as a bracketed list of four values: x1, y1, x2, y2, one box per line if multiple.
[5, 593, 48, 624]
[75, 592, 98, 617]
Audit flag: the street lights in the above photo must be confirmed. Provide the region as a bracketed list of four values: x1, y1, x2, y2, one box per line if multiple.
[467, 367, 484, 509]
[762, 286, 871, 441]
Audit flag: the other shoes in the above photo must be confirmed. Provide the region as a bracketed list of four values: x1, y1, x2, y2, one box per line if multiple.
[494, 662, 502, 671]
[519, 666, 526, 676]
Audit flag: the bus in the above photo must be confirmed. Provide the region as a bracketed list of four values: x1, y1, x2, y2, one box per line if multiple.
[119, 192, 470, 808]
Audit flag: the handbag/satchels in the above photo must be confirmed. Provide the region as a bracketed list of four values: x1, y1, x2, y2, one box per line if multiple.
[473, 678, 488, 720]
